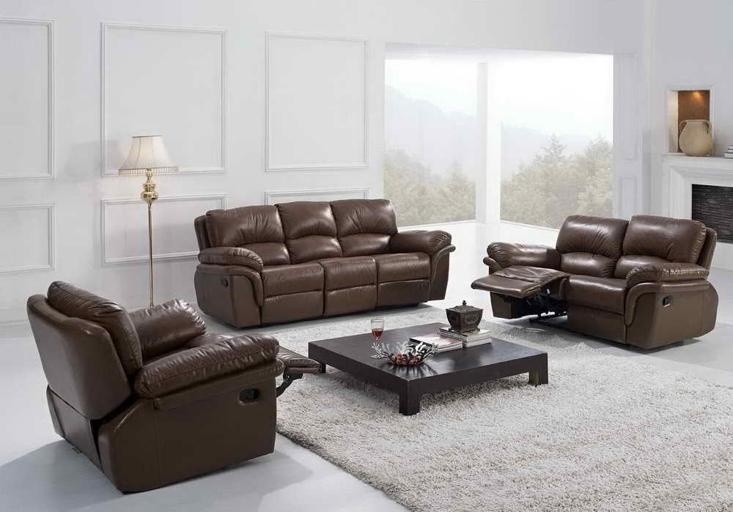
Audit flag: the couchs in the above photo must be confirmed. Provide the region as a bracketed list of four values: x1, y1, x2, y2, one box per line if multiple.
[193, 198, 457, 330]
[468, 212, 720, 353]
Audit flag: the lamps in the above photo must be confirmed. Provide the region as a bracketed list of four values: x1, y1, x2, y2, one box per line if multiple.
[117, 134, 180, 309]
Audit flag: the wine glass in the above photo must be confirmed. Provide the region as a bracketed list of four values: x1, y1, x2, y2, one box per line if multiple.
[371, 317, 385, 351]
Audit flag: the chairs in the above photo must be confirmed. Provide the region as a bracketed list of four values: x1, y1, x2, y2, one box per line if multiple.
[25, 280, 322, 495]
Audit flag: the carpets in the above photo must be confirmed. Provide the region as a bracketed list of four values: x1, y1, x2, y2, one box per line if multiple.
[263, 306, 732, 512]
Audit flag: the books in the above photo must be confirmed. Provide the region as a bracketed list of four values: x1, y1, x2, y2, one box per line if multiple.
[438, 334, 491, 348]
[438, 325, 492, 339]
[408, 341, 463, 355]
[721, 145, 732, 159]
[408, 332, 462, 348]
